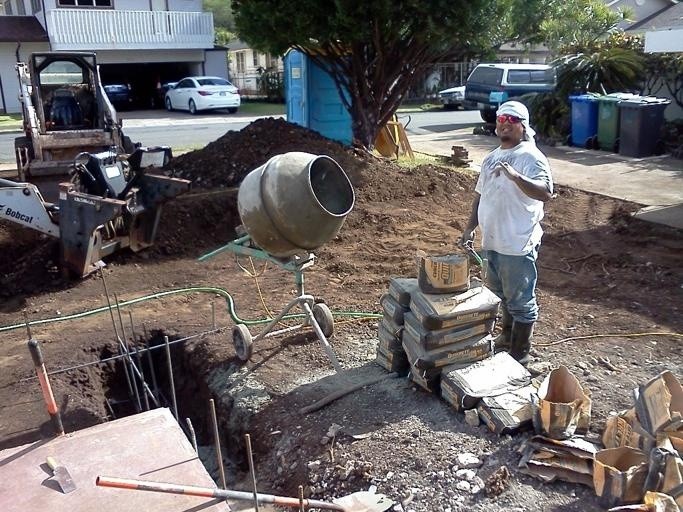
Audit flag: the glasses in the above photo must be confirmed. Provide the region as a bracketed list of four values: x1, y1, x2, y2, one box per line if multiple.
[495, 115, 527, 125]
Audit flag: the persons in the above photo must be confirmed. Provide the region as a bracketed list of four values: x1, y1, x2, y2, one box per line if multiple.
[461, 101, 554, 369]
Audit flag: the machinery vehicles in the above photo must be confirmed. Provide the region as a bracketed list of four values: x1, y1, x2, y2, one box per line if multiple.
[12, 50, 133, 205]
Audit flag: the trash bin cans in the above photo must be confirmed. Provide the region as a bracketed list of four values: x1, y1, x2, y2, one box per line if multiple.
[617, 94, 672, 158]
[593, 94, 621, 154]
[566, 93, 598, 150]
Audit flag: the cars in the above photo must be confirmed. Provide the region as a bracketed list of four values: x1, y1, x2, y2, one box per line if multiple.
[164, 76, 241, 114]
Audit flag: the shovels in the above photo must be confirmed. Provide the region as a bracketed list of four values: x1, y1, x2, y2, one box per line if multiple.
[47, 456, 77, 494]
[97, 475, 393, 512]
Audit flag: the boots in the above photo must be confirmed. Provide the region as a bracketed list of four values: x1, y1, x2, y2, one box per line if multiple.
[492, 308, 512, 349]
[509, 321, 534, 362]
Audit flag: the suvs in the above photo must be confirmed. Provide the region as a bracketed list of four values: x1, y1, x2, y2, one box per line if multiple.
[439, 85, 465, 105]
[461, 62, 556, 123]
